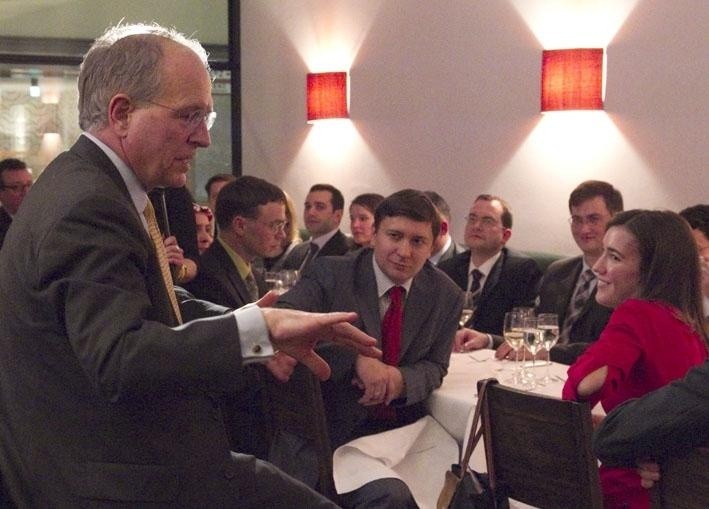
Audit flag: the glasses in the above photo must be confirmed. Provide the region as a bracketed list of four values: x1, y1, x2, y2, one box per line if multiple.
[144, 99, 217, 131]
[250, 216, 287, 235]
[568, 213, 610, 225]
[464, 215, 506, 230]
[3, 181, 32, 194]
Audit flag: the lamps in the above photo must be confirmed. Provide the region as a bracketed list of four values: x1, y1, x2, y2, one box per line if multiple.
[305, 69, 351, 121]
[538, 45, 606, 113]
[27, 75, 43, 99]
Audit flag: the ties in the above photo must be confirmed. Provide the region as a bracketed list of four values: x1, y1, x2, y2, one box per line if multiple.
[373, 286, 404, 421]
[468, 269, 482, 312]
[144, 199, 184, 325]
[245, 274, 259, 304]
[302, 243, 318, 270]
[558, 269, 596, 345]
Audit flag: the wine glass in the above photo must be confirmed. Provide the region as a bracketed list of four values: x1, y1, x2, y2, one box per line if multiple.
[538, 313, 559, 386]
[503, 312, 528, 382]
[457, 291, 474, 354]
[264, 268, 298, 294]
[523, 316, 544, 381]
[515, 308, 534, 377]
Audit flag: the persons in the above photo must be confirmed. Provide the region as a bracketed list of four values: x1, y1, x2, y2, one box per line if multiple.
[1, 23, 383, 509]
[150, 174, 384, 431]
[561, 204, 708, 509]
[1, 158, 33, 249]
[426, 180, 625, 362]
[275, 188, 467, 508]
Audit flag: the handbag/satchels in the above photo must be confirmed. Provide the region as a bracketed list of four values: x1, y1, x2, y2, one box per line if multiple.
[437, 462, 511, 509]
